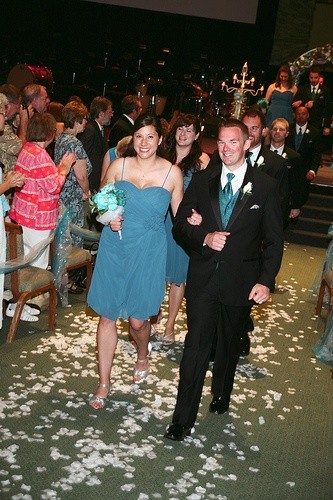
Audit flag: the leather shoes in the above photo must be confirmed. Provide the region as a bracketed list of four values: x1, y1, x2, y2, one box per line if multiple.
[209, 394, 231, 415]
[67, 283, 84, 294]
[164, 422, 192, 440]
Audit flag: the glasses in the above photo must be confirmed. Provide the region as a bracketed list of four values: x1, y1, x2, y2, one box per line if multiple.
[83, 118, 88, 121]
[0, 112, 7, 116]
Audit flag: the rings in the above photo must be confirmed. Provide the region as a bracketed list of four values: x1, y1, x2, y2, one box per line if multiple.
[193, 220, 195, 222]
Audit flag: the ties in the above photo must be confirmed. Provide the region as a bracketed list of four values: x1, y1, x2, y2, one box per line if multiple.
[298, 128, 304, 147]
[311, 87, 316, 101]
[274, 150, 278, 155]
[224, 173, 235, 198]
[246, 152, 254, 167]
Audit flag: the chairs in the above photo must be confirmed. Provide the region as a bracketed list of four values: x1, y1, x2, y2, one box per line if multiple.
[2, 216, 93, 344]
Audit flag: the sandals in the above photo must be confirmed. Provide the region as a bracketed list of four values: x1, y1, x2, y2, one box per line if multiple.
[134, 357, 150, 384]
[90, 381, 113, 410]
[163, 328, 174, 347]
[148, 312, 161, 336]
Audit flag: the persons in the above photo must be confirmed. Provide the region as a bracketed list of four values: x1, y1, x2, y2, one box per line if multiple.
[44, 94, 180, 251]
[261, 65, 298, 140]
[164, 119, 286, 442]
[284, 106, 323, 222]
[0, 84, 22, 134]
[0, 93, 26, 329]
[255, 117, 307, 257]
[51, 101, 94, 306]
[292, 69, 333, 168]
[87, 112, 202, 408]
[318, 75, 326, 86]
[0, 84, 75, 322]
[205, 110, 288, 357]
[149, 114, 211, 345]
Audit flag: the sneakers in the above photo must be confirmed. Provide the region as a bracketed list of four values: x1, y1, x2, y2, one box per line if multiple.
[6, 303, 39, 321]
[23, 303, 40, 315]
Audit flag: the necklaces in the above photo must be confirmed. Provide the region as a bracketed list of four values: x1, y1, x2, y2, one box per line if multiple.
[136, 163, 155, 177]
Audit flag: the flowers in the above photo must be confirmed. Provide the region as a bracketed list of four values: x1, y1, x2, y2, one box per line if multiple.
[257, 97, 268, 109]
[88, 182, 127, 241]
[254, 155, 265, 168]
[238, 181, 253, 200]
[282, 152, 289, 160]
